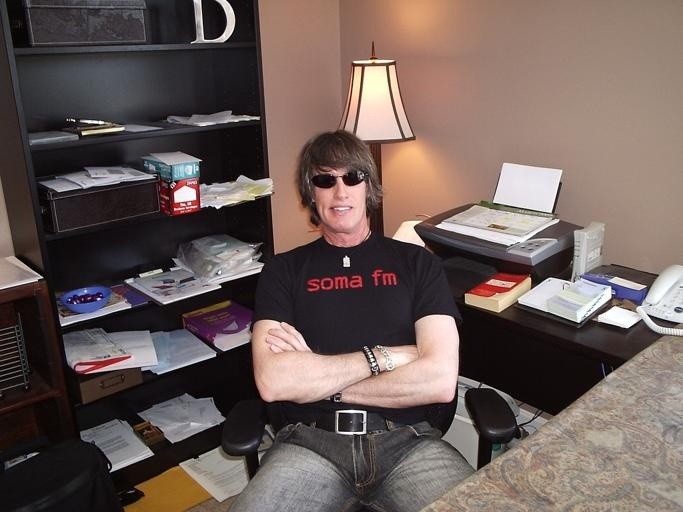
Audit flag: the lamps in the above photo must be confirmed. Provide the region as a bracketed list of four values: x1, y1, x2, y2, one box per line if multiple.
[336, 41, 415, 236]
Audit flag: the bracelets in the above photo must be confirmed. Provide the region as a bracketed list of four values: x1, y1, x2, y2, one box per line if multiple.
[357, 341, 381, 377]
[374, 341, 395, 372]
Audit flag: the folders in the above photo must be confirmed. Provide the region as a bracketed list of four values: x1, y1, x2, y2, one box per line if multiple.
[583, 272, 648, 302]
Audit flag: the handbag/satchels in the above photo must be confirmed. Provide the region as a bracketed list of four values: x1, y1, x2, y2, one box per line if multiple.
[1, 434, 144, 512]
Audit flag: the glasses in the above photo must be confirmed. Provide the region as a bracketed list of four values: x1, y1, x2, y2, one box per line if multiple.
[310, 169, 367, 189]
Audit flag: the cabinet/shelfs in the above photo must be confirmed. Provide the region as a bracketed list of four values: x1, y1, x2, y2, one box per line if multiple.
[0, 254, 72, 452]
[0, 0, 275, 491]
[432, 254, 680, 416]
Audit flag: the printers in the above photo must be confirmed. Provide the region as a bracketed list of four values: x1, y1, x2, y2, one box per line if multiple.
[414, 162, 584, 303]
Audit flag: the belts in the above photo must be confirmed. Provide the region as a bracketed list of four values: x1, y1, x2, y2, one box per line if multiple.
[301, 406, 404, 436]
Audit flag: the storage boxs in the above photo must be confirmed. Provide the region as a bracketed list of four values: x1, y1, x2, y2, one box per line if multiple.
[6, 0, 147, 45]
[35, 164, 161, 235]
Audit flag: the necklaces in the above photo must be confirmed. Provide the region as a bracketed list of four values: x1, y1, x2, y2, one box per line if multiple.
[314, 228, 375, 272]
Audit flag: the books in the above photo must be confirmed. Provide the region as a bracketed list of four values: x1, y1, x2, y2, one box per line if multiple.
[30, 111, 259, 144]
[56, 261, 264, 326]
[80, 394, 227, 472]
[462, 269, 535, 314]
[63, 301, 254, 375]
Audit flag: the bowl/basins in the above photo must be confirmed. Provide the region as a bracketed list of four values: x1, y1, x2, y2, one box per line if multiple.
[62, 287, 112, 313]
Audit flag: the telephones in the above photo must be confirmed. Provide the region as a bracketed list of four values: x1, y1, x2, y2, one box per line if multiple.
[641, 264, 683, 323]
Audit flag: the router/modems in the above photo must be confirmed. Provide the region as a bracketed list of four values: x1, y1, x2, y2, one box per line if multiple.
[571, 221, 605, 284]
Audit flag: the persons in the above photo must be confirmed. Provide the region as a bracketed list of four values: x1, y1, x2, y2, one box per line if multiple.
[227, 129, 479, 511]
